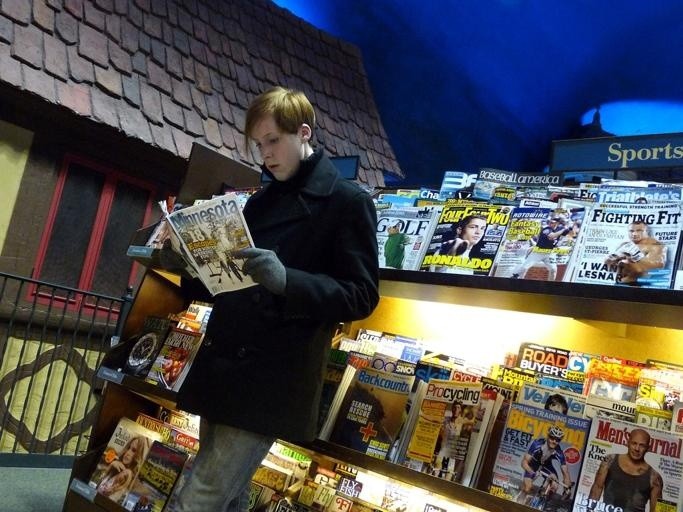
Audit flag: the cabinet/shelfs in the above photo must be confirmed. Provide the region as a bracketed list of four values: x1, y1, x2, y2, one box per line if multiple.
[60, 141, 682, 511]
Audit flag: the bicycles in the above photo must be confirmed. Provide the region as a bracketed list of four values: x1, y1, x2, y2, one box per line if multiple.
[513, 455, 575, 512]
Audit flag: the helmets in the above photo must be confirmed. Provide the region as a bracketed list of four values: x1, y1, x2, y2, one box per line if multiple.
[452, 399, 462, 408]
[547, 426, 565, 440]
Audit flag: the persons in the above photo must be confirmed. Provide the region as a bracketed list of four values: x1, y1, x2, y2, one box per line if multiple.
[173, 86, 379, 512]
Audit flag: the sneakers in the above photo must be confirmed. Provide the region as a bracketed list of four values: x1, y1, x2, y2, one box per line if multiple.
[511, 274, 521, 279]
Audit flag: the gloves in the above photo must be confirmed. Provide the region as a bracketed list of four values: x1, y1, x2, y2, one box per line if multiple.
[158, 238, 188, 276]
[622, 241, 643, 263]
[608, 242, 627, 262]
[234, 248, 288, 295]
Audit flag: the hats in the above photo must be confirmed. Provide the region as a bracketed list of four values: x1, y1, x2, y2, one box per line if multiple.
[386, 220, 398, 228]
[549, 216, 561, 223]
